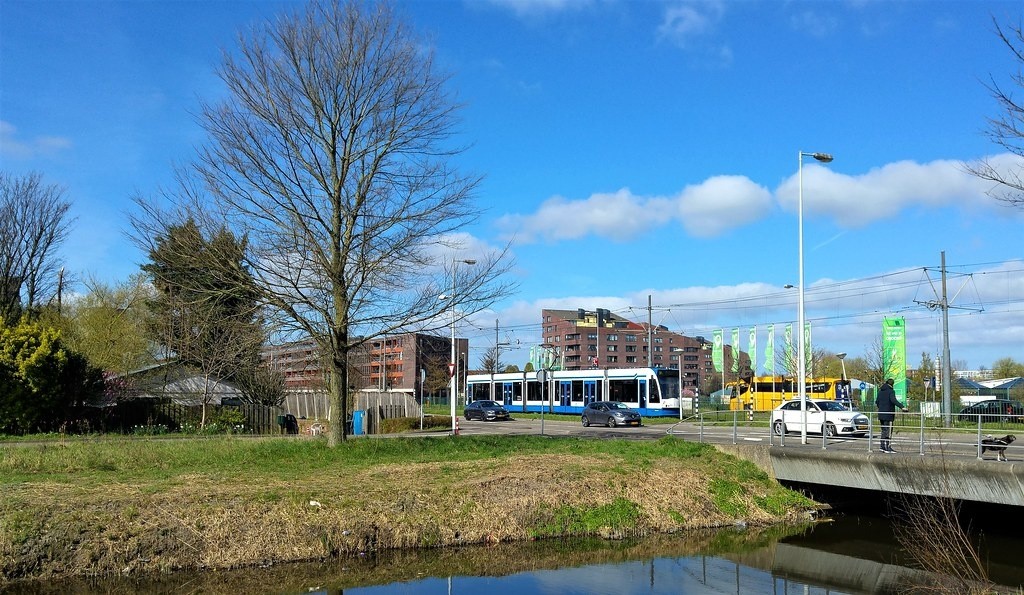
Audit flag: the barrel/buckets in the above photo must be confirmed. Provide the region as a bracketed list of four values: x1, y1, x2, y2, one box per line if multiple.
[353, 411, 364, 435]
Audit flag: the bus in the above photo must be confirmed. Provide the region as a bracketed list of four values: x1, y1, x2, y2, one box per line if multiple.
[466, 367, 686, 417]
[724, 376, 851, 411]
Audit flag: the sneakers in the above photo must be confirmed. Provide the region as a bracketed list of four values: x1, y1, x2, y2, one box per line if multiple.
[884, 447, 897, 454]
[879, 446, 886, 451]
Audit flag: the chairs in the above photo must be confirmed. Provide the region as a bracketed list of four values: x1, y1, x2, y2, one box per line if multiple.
[310, 423, 321, 436]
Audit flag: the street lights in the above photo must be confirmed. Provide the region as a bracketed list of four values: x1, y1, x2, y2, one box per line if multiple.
[461, 352, 466, 405]
[784, 151, 835, 444]
[836, 352, 853, 410]
[438, 258, 477, 435]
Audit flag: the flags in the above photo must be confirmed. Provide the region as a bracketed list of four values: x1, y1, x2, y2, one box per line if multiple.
[804, 322, 811, 377]
[748, 327, 755, 371]
[711, 330, 722, 373]
[731, 328, 738, 372]
[785, 324, 791, 373]
[765, 326, 773, 372]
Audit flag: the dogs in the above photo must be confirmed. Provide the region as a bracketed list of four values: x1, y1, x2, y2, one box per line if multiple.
[974, 435, 1017, 462]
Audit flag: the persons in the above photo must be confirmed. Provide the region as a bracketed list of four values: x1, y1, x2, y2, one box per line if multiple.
[875, 379, 908, 453]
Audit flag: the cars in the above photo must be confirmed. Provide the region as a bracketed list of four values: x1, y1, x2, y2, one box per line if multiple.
[464, 400, 510, 422]
[581, 401, 641, 429]
[958, 399, 1024, 423]
[768, 399, 870, 439]
